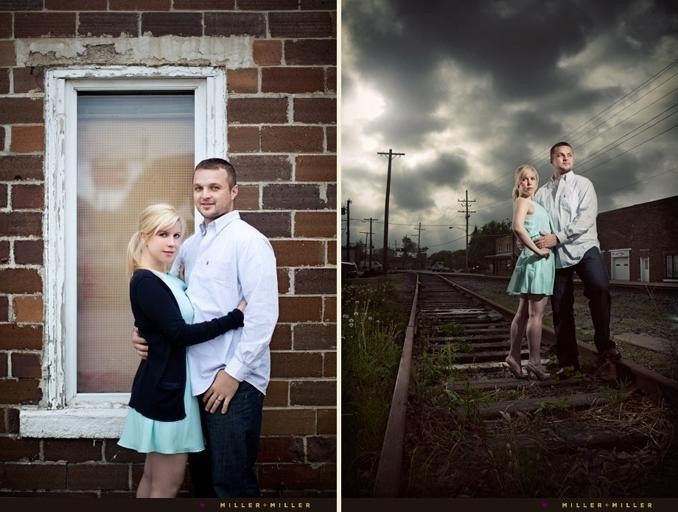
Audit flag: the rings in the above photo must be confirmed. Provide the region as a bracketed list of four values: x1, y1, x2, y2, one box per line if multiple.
[216, 398, 222, 401]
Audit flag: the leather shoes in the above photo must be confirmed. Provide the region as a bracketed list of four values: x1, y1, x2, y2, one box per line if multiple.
[600, 347, 622, 360]
[556, 361, 580, 376]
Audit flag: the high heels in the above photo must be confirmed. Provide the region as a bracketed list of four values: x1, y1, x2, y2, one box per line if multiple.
[502, 357, 528, 380]
[526, 360, 551, 381]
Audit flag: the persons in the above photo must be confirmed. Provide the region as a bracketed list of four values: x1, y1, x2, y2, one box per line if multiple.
[502, 165, 555, 381]
[116, 203, 248, 498]
[514, 141, 622, 379]
[131, 157, 280, 498]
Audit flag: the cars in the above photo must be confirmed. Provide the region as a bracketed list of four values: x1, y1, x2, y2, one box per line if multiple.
[342, 261, 358, 279]
[431, 261, 455, 272]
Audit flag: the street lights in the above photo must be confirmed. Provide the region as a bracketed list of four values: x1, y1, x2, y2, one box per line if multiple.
[449, 227, 468, 273]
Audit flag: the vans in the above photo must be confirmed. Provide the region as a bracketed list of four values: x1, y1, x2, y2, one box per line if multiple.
[360, 261, 383, 273]
